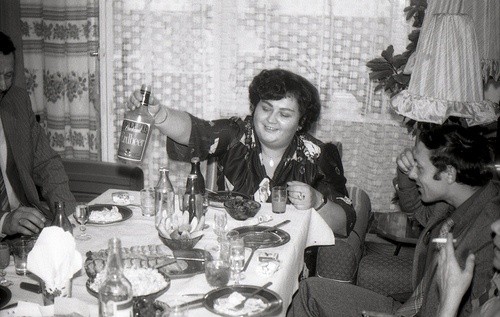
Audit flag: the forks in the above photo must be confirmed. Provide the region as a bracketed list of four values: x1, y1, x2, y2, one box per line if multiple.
[233, 281, 273, 311]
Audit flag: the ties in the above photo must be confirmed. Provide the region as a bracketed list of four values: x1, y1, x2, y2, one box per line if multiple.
[0, 167, 11, 212]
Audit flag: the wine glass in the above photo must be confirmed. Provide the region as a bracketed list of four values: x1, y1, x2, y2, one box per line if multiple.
[213, 211, 246, 286]
[0, 242, 12, 287]
[74, 201, 92, 240]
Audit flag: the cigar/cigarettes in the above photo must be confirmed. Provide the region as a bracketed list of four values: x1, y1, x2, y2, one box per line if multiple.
[432, 238, 456, 243]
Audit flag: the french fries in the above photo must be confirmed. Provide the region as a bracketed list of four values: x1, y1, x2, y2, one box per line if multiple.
[154, 209, 205, 240]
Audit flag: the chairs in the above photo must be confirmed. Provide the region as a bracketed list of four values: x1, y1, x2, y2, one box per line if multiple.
[315, 184, 419, 298]
[61, 159, 143, 203]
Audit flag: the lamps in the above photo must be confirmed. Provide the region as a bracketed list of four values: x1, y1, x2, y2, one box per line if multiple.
[389, 12, 499, 128]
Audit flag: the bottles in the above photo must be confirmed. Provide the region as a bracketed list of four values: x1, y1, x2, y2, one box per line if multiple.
[52, 200, 74, 237]
[185, 156, 205, 222]
[154, 166, 174, 228]
[99, 238, 134, 317]
[116, 85, 153, 165]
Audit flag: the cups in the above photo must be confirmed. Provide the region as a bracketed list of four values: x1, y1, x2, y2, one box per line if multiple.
[41, 277, 72, 306]
[205, 241, 231, 285]
[13, 239, 33, 274]
[272, 186, 287, 213]
[140, 187, 155, 216]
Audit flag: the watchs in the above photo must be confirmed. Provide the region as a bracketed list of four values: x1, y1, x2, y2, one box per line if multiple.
[315, 194, 327, 211]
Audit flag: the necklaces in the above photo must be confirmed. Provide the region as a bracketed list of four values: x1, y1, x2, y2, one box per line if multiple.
[270, 160, 274, 167]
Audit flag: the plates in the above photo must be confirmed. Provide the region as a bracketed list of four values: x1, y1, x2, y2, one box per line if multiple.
[84, 245, 285, 317]
[228, 225, 290, 248]
[207, 191, 251, 204]
[0, 285, 12, 308]
[73, 204, 132, 227]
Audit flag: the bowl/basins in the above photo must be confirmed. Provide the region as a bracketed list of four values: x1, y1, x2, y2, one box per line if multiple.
[223, 197, 262, 220]
[158, 233, 203, 248]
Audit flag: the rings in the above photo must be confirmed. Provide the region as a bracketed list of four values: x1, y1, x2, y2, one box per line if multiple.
[298, 193, 302, 199]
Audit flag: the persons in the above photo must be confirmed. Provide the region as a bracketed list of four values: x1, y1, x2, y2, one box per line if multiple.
[287, 125, 500, 316]
[437, 219, 500, 317]
[0, 32, 79, 253]
[126, 69, 356, 239]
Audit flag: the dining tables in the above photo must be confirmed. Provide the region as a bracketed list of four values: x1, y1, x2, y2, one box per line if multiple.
[0, 189, 336, 317]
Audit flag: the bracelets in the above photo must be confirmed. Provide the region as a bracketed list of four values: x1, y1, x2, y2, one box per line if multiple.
[156, 110, 168, 124]
